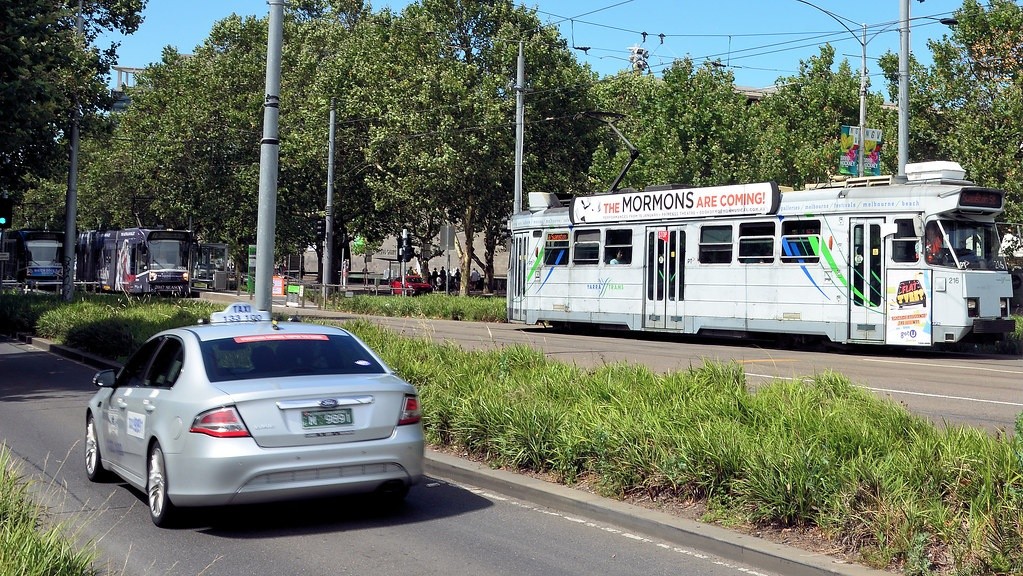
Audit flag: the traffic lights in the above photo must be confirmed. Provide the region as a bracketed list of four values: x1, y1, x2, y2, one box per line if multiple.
[405, 247, 415, 261]
[0, 198, 12, 229]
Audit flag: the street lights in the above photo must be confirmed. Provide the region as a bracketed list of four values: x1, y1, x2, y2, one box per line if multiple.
[799, 1, 959, 178]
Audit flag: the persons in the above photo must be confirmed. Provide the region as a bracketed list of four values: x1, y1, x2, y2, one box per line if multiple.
[470, 268, 480, 291]
[610, 248, 626, 264]
[428, 266, 461, 292]
[407, 266, 418, 276]
[915, 227, 948, 262]
[154, 254, 167, 264]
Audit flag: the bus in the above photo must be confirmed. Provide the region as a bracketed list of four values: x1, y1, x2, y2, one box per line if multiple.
[76, 229, 200, 298]
[506, 161, 1016, 347]
[1, 230, 68, 292]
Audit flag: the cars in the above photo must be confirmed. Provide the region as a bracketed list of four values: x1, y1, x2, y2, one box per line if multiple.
[390, 276, 433, 297]
[84, 302, 426, 528]
